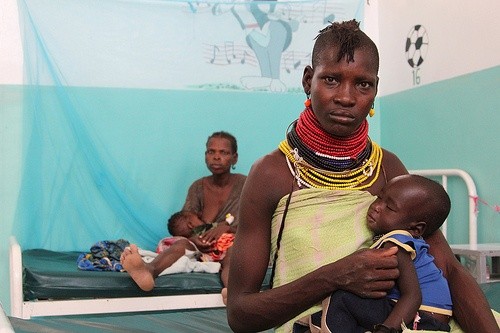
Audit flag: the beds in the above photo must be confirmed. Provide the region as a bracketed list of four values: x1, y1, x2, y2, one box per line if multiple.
[0, 278, 500, 333]
[10, 169, 477, 320]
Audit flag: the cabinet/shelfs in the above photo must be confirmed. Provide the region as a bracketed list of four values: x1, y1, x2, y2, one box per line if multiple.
[449, 243, 500, 281]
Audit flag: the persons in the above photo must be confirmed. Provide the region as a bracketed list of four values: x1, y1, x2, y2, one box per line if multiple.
[225, 17, 499, 332]
[119, 129, 251, 306]
[167, 208, 237, 261]
[292, 172, 455, 332]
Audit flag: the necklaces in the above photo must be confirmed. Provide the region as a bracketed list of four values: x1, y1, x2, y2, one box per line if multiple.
[372, 232, 424, 241]
[277, 100, 384, 194]
